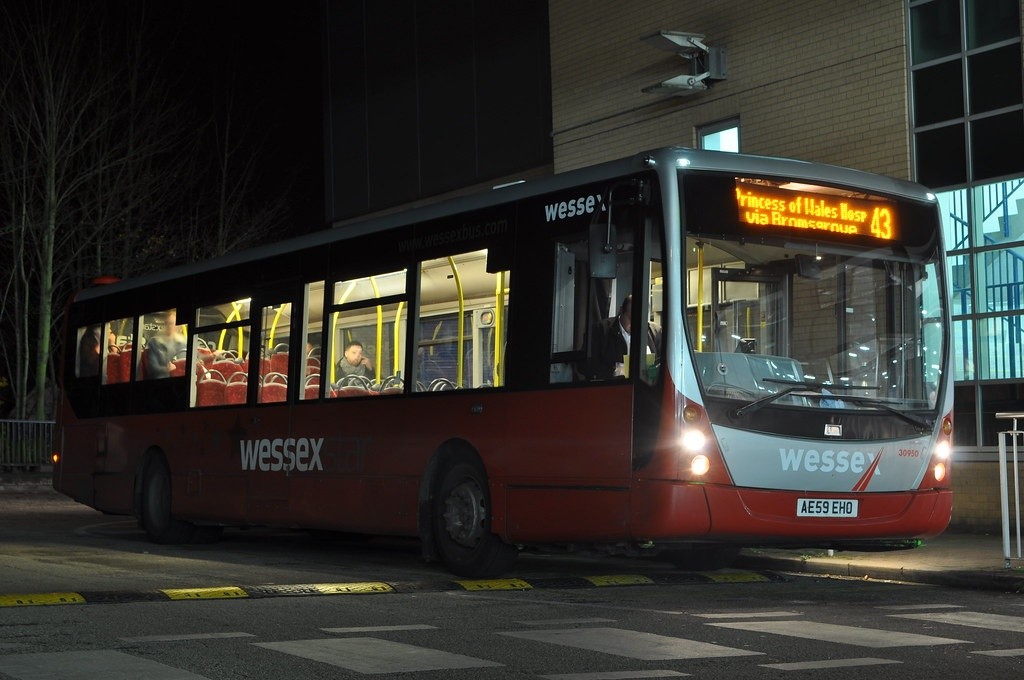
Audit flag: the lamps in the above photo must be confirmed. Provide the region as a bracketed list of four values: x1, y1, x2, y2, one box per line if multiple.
[639, 30, 729, 97]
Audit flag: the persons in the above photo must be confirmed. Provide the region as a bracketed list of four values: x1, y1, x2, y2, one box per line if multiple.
[580, 294, 662, 378]
[142, 310, 226, 379]
[334, 341, 376, 387]
[307, 342, 321, 362]
[79, 325, 118, 376]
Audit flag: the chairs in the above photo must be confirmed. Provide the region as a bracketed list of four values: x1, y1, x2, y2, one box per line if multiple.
[107, 338, 495, 406]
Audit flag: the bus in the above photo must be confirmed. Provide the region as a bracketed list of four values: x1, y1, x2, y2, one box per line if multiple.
[50, 147, 955, 581]
